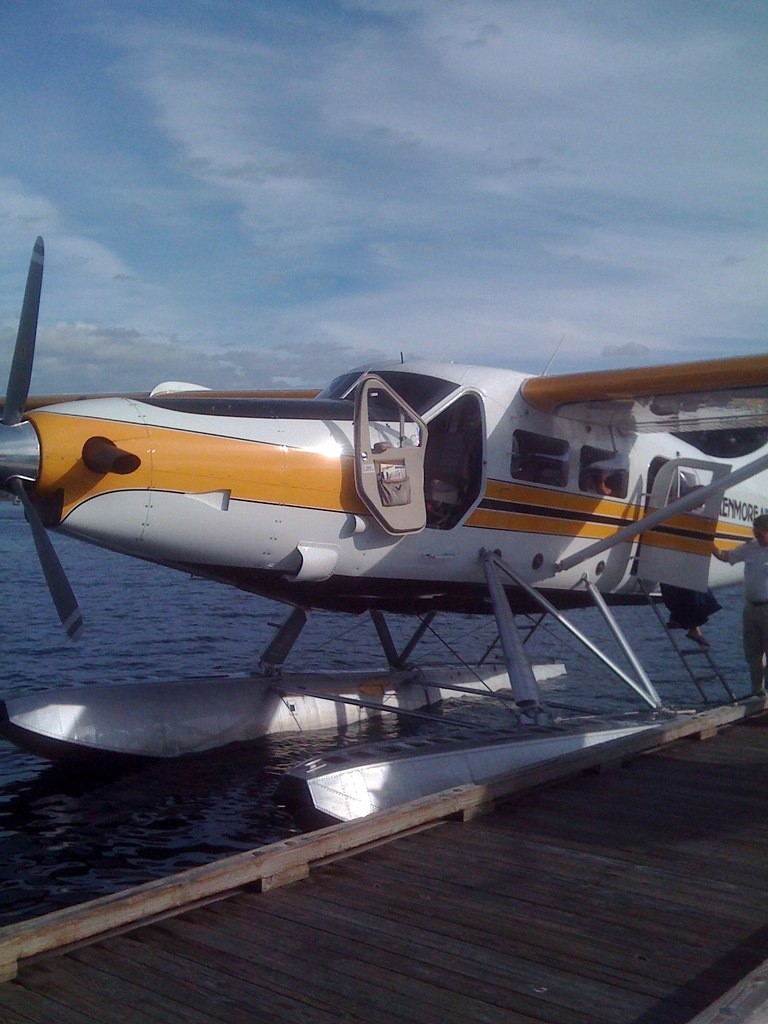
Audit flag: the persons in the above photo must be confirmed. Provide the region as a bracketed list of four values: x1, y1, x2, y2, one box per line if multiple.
[712, 513, 768, 694]
[660, 484, 722, 646]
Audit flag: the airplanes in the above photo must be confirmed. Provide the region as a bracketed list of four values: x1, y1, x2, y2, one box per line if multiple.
[0, 230, 768, 831]
